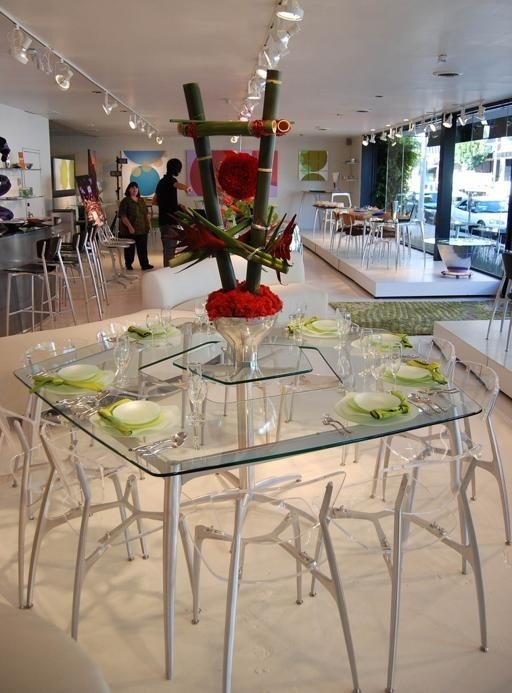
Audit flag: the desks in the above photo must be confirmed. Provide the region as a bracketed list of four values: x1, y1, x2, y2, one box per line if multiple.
[422, 236, 496, 278]
[299, 188, 352, 229]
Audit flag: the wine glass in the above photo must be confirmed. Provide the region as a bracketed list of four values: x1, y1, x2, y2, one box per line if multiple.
[146, 299, 307, 346]
[332, 308, 381, 377]
[113, 334, 132, 385]
[369, 343, 402, 396]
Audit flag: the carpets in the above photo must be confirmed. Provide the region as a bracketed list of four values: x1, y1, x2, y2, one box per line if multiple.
[326, 298, 511, 336]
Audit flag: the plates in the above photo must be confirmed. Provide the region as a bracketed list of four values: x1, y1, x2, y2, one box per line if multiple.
[311, 320, 335, 330]
[58, 363, 99, 380]
[394, 367, 430, 378]
[1, 216, 44, 227]
[112, 398, 160, 423]
[355, 392, 401, 412]
[368, 334, 402, 344]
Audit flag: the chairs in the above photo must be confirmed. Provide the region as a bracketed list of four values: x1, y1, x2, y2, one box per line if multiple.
[95, 311, 209, 351]
[313, 443, 490, 693]
[379, 361, 511, 547]
[485, 248, 511, 353]
[0, 214, 137, 336]
[175, 471, 362, 693]
[0, 407, 133, 610]
[13, 337, 148, 485]
[25, 424, 201, 641]
[340, 335, 457, 498]
[312, 201, 426, 272]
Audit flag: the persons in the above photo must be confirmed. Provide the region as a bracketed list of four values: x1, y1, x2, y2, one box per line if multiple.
[118, 182, 154, 270]
[152, 158, 192, 268]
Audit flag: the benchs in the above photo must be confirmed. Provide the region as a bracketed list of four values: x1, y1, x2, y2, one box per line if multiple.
[141, 246, 326, 382]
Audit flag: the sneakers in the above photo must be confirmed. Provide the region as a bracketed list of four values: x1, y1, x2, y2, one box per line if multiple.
[141, 264, 154, 270]
[126, 265, 133, 270]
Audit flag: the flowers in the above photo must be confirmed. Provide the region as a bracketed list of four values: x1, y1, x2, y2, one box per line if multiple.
[166, 64, 305, 354]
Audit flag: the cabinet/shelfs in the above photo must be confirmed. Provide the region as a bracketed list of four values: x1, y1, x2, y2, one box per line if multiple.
[342, 160, 360, 182]
[1, 162, 45, 201]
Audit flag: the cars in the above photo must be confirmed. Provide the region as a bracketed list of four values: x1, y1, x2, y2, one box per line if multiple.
[399, 181, 512, 242]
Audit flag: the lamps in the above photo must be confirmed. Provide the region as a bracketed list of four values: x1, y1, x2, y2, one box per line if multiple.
[360, 104, 491, 146]
[227, 0, 309, 144]
[6, 23, 162, 147]
